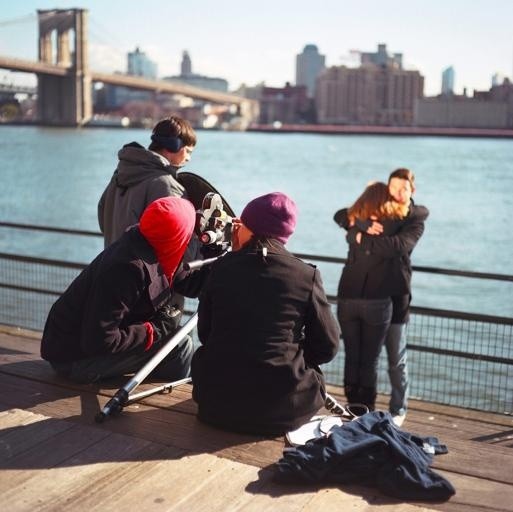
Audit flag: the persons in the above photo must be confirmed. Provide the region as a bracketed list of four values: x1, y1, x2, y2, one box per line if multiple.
[37, 196, 195, 384]
[93, 116, 197, 312]
[170, 189, 341, 436]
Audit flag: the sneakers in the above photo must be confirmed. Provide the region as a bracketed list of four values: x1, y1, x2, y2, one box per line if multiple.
[392, 409, 407, 428]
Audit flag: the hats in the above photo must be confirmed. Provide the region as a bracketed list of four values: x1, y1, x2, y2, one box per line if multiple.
[239, 190, 299, 244]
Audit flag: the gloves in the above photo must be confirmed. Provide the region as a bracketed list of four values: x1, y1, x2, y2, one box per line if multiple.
[149, 302, 183, 344]
